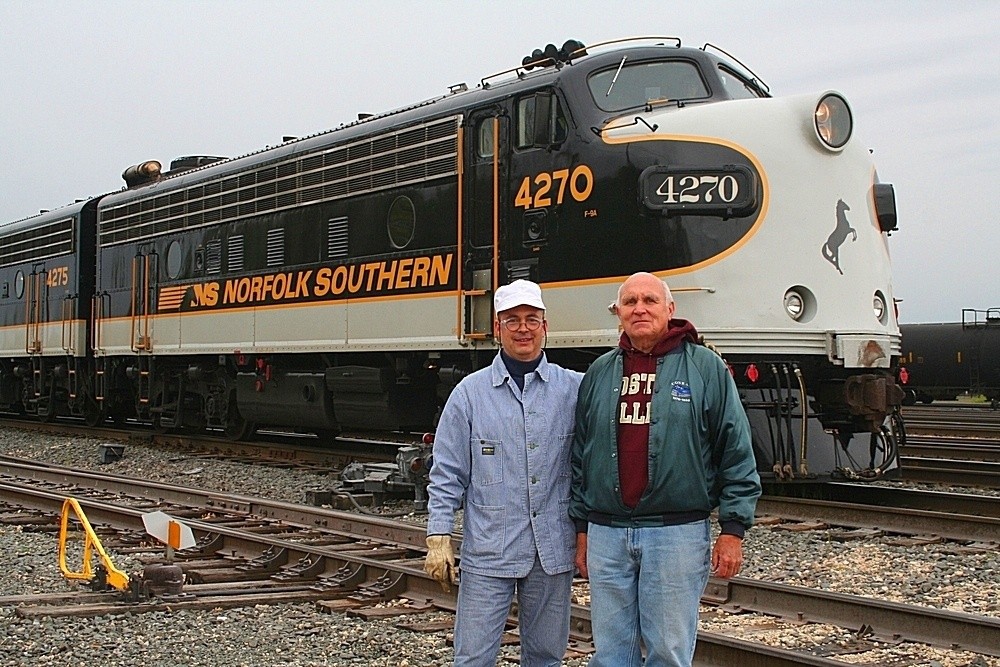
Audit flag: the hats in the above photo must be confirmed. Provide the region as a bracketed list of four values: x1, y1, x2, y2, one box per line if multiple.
[493, 279, 546, 317]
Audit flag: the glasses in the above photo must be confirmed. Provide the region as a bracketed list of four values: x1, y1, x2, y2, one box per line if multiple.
[499, 316, 544, 332]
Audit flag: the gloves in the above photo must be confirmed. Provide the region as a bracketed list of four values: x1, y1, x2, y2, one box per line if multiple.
[425, 535, 456, 593]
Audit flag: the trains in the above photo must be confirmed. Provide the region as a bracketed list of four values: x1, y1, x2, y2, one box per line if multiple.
[0, 36, 910, 487]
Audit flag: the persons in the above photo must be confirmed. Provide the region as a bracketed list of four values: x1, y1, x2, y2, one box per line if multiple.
[567, 271, 763, 667]
[426, 279, 726, 667]
